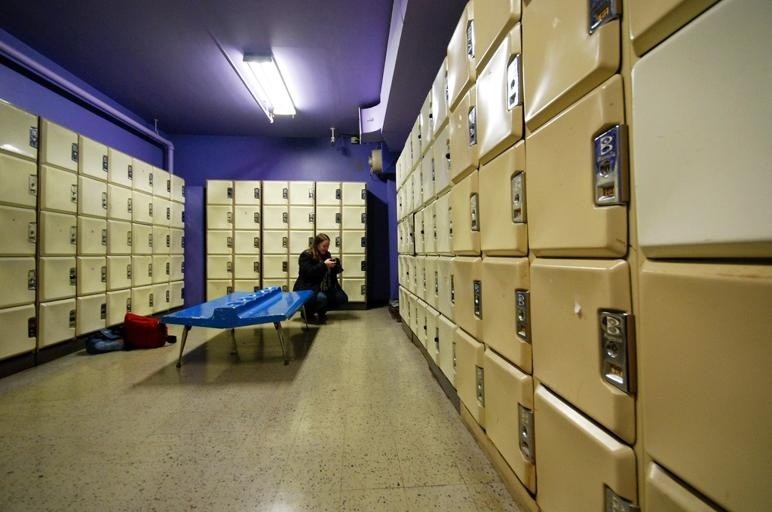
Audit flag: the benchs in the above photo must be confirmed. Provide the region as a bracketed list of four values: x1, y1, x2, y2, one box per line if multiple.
[160, 285, 315, 368]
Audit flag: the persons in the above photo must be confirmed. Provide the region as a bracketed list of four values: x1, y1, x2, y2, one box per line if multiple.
[292, 233, 348, 324]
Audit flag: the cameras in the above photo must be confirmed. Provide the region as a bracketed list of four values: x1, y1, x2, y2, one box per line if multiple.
[330, 259, 343, 273]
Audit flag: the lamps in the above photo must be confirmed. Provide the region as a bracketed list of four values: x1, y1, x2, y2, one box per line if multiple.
[242, 52, 297, 116]
[330, 127, 336, 144]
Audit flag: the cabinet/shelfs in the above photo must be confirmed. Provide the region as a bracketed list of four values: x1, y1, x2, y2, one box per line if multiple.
[395, 0, 772, 512]
[204, 179, 367, 311]
[0, 99, 186, 380]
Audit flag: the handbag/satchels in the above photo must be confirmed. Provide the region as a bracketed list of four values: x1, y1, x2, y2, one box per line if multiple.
[120, 313, 177, 349]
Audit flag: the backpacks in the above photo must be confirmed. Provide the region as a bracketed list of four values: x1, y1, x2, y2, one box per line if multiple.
[84, 328, 124, 354]
